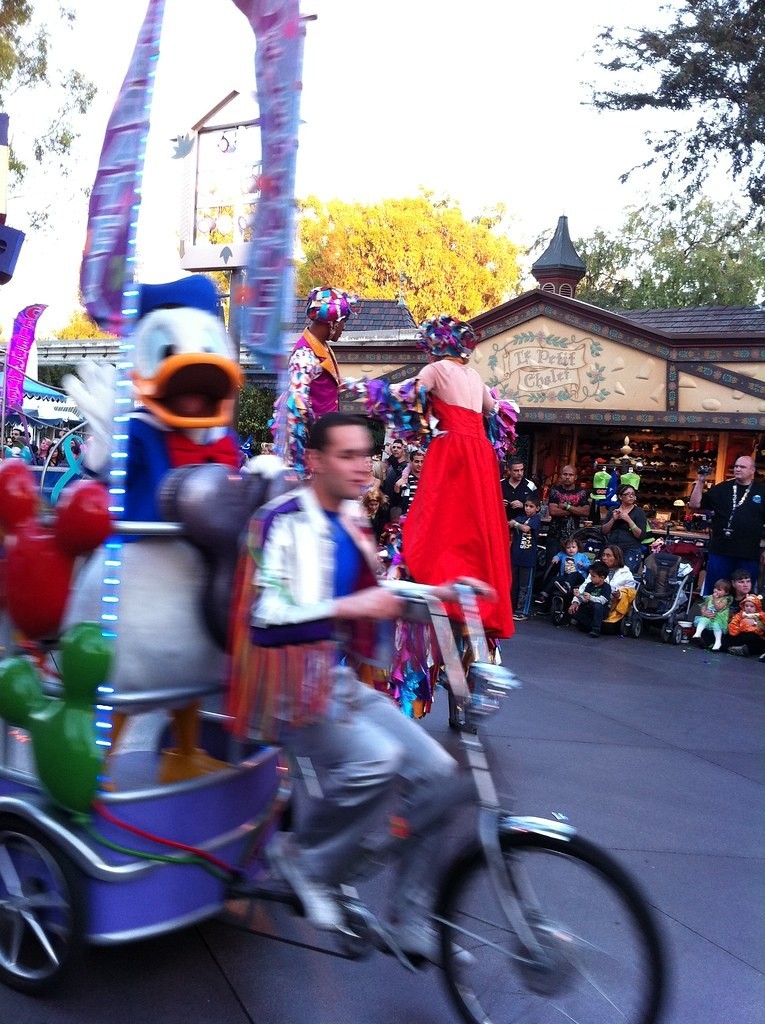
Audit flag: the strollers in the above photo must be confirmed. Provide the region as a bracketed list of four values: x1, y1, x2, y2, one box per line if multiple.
[628, 527, 714, 642]
[547, 524, 609, 627]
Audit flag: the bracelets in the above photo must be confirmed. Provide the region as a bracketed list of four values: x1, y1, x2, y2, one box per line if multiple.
[629, 524, 637, 531]
[566, 504, 570, 511]
[514, 521, 519, 535]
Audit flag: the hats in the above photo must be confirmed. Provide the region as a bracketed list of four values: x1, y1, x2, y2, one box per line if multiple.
[14, 436, 28, 445]
[303, 285, 354, 322]
[415, 316, 481, 359]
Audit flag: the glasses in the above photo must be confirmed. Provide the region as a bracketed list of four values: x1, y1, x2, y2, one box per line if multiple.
[621, 493, 635, 497]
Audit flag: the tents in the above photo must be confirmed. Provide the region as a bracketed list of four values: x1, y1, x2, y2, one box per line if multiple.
[0, 371, 66, 403]
[5, 409, 83, 429]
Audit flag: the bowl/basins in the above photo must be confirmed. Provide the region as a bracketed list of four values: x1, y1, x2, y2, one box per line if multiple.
[583, 521, 593, 527]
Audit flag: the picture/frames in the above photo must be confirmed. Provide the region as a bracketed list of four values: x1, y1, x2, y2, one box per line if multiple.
[655, 511, 672, 521]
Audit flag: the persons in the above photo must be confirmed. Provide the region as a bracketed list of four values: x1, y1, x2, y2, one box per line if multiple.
[345, 317, 517, 665]
[272, 286, 351, 480]
[0, 429, 93, 468]
[234, 412, 495, 966]
[261, 439, 765, 663]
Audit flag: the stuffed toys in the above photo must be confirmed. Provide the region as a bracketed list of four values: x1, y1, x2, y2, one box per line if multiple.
[65, 273, 243, 791]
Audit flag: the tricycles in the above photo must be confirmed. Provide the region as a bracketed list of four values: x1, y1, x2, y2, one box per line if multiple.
[0, 580, 671, 1024]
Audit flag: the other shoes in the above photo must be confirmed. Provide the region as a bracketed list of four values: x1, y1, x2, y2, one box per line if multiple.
[534, 594, 545, 603]
[589, 629, 599, 637]
[555, 581, 569, 594]
[373, 916, 475, 965]
[266, 831, 343, 930]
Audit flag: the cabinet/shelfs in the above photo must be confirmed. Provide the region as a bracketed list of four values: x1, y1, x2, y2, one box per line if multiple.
[576, 432, 691, 500]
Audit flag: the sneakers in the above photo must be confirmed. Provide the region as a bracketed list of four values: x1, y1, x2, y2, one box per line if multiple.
[514, 614, 529, 620]
